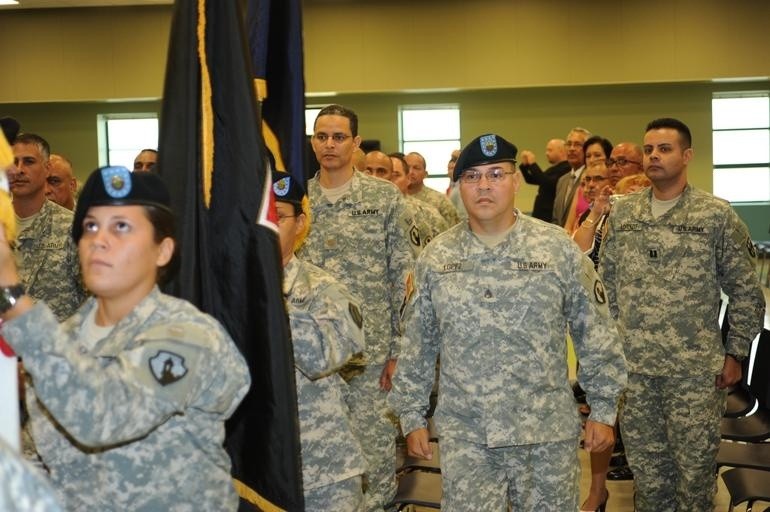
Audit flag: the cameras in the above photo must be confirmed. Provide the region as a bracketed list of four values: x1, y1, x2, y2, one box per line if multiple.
[609, 194, 626, 206]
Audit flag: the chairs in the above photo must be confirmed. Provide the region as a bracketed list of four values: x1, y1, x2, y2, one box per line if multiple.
[702, 236, 770, 511]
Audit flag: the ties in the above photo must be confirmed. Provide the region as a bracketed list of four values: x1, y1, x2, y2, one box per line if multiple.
[564, 174, 576, 211]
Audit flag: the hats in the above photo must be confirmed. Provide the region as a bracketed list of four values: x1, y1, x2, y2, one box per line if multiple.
[70, 165, 174, 247]
[452, 132, 518, 183]
[271, 170, 306, 216]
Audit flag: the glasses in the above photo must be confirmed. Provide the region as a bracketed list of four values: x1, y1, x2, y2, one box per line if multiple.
[276, 214, 298, 225]
[458, 169, 514, 182]
[604, 159, 639, 167]
[316, 134, 352, 144]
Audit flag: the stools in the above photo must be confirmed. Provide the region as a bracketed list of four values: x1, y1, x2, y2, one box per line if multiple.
[395, 354, 443, 512]
